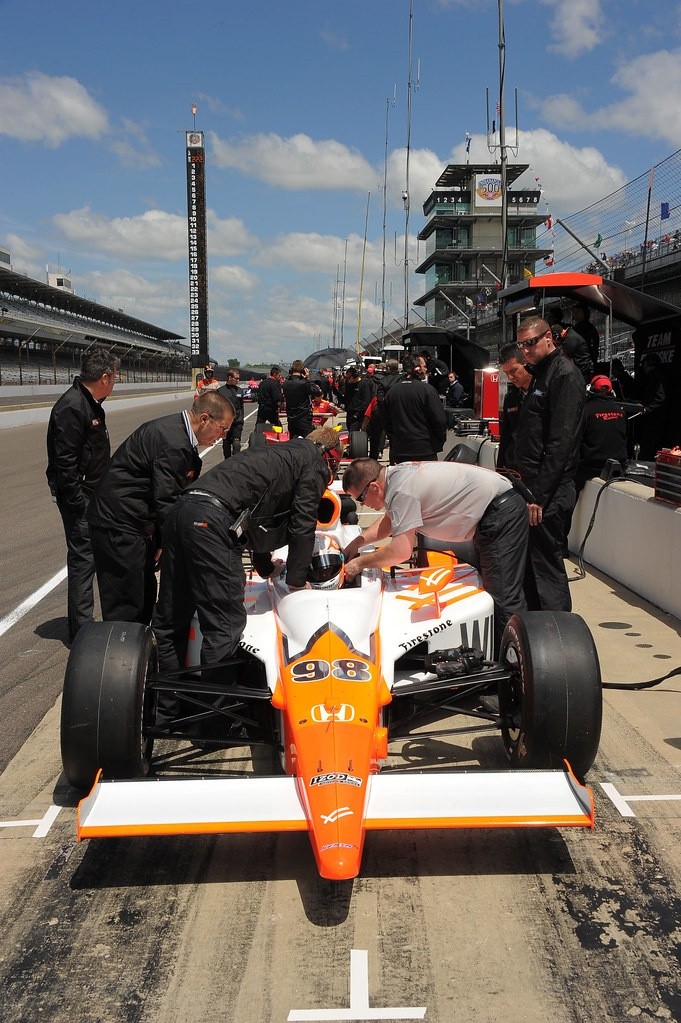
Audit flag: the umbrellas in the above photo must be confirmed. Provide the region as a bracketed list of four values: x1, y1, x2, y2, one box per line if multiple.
[304, 345, 363, 370]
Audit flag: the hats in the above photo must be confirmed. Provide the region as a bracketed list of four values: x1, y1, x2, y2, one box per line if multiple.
[639, 353, 662, 367]
[590, 374, 613, 395]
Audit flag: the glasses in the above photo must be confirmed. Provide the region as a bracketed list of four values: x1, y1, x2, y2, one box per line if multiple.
[202, 411, 231, 433]
[346, 375, 352, 379]
[356, 479, 377, 503]
[232, 376, 239, 380]
[516, 329, 550, 348]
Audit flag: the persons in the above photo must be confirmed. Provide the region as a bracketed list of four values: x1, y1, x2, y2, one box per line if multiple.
[496, 316, 587, 611]
[153, 427, 343, 741]
[281, 360, 323, 439]
[45, 347, 123, 645]
[369, 350, 449, 463]
[318, 359, 402, 462]
[306, 534, 346, 590]
[550, 322, 595, 383]
[250, 367, 283, 445]
[447, 372, 464, 410]
[87, 392, 237, 621]
[581, 227, 681, 277]
[342, 458, 530, 717]
[194, 364, 223, 402]
[627, 355, 669, 456]
[216, 369, 245, 459]
[562, 375, 631, 560]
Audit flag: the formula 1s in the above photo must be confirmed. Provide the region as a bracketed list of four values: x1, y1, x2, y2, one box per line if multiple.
[246, 413, 369, 482]
[58, 479, 605, 880]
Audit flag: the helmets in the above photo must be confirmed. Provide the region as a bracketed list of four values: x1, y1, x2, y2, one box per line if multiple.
[304, 532, 345, 590]
[204, 365, 214, 373]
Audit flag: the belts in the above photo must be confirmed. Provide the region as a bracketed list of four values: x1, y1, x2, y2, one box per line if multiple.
[480, 487, 520, 521]
[181, 490, 239, 523]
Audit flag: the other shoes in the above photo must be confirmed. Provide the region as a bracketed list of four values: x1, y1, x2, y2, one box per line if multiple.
[480, 694, 499, 712]
